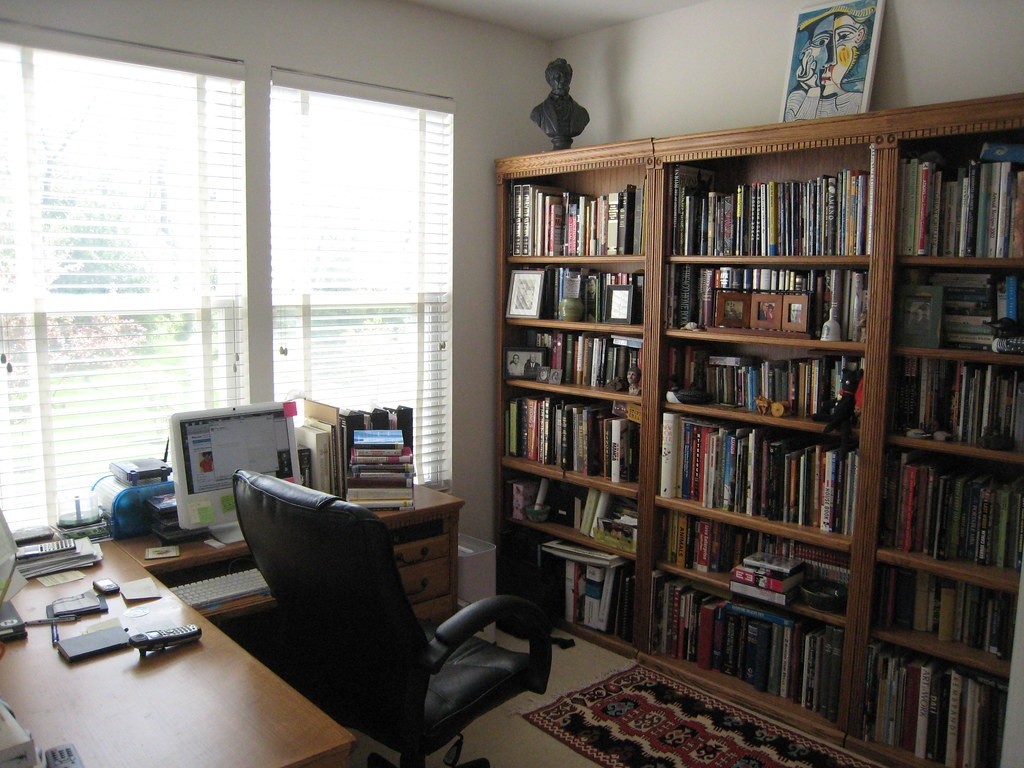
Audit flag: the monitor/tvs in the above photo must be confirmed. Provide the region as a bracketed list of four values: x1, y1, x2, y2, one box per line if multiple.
[170, 402, 301, 545]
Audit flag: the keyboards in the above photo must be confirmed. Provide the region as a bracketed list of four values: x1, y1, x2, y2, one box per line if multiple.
[170, 568, 270, 609]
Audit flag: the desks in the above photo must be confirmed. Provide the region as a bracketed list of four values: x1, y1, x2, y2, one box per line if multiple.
[0, 485, 466, 768]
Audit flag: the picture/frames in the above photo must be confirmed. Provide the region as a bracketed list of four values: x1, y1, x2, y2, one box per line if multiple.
[712, 288, 812, 341]
[778, 0, 886, 124]
[505, 270, 545, 319]
[603, 284, 634, 325]
[503, 346, 548, 379]
[891, 286, 944, 348]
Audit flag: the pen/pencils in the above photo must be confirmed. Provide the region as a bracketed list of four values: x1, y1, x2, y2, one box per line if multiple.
[51, 620, 60, 644]
[24, 614, 82, 625]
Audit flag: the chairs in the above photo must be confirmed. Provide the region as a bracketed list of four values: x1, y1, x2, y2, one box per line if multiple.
[232, 468, 553, 768]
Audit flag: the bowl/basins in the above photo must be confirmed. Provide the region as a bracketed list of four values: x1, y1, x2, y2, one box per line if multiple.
[799, 580, 848, 613]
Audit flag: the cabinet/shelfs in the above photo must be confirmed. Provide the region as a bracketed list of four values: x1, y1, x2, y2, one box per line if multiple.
[497, 90, 1024, 768]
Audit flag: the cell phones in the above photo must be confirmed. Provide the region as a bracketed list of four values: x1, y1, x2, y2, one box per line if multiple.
[92, 579, 121, 595]
[129, 624, 200, 651]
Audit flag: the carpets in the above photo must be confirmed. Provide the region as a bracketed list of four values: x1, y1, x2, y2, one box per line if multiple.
[519, 661, 883, 768]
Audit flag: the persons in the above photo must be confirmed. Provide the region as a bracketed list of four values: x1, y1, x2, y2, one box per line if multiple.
[530, 58, 589, 152]
[586, 276, 596, 299]
[758, 303, 767, 320]
[525, 352, 539, 377]
[766, 304, 775, 321]
[628, 367, 641, 395]
[508, 354, 524, 377]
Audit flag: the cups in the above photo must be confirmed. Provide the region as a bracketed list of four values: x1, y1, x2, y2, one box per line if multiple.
[559, 298, 585, 322]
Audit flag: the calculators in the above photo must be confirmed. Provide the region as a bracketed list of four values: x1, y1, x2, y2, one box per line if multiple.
[16, 538, 77, 560]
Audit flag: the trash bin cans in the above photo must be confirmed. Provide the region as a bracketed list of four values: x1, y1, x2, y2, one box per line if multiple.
[456, 533, 498, 645]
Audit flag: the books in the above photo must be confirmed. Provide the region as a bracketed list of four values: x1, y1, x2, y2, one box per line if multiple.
[648, 141, 872, 724]
[858, 141, 1024, 768]
[342, 429, 415, 512]
[506, 180, 646, 644]
